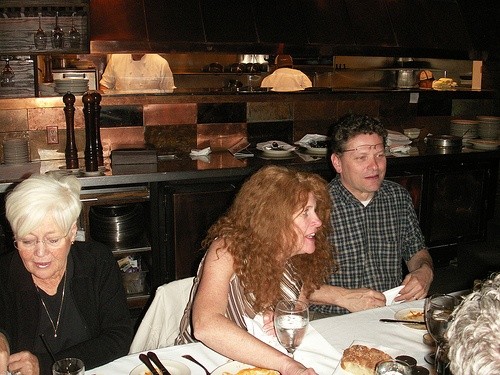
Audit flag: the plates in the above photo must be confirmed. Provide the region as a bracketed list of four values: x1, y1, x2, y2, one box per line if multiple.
[257, 147, 296, 155]
[54, 79, 89, 95]
[210, 361, 255, 375]
[450, 115, 500, 147]
[129, 359, 191, 375]
[395, 308, 427, 330]
[3, 139, 29, 164]
[59, 165, 105, 176]
[308, 146, 327, 153]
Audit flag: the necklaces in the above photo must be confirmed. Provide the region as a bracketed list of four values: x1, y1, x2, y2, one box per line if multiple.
[32, 270, 66, 338]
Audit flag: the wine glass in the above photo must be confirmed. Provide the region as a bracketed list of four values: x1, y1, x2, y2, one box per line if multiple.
[2, 56, 15, 82]
[52, 12, 64, 49]
[68, 14, 80, 49]
[424, 293, 460, 365]
[274, 299, 309, 359]
[34, 12, 47, 49]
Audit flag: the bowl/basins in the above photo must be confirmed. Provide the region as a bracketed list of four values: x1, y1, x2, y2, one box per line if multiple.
[432, 135, 462, 147]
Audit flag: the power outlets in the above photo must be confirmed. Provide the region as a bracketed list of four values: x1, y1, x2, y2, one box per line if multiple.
[47, 126, 59, 144]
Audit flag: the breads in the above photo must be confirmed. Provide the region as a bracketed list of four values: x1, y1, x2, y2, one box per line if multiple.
[341, 345, 394, 375]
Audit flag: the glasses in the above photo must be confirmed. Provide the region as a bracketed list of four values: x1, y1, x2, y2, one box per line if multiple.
[338, 142, 387, 154]
[13, 225, 71, 250]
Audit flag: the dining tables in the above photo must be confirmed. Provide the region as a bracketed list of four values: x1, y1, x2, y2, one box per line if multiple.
[82, 300, 441, 375]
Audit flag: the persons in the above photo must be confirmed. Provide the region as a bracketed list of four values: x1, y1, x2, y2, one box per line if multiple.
[261, 54, 312, 91]
[0, 171, 133, 375]
[443, 273, 499, 375]
[303, 111, 433, 316]
[174, 164, 334, 375]
[99, 54, 174, 91]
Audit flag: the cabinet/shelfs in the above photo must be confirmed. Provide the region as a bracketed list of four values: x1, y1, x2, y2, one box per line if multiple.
[158, 151, 500, 299]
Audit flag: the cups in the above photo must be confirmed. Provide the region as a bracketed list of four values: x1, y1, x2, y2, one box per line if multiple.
[52, 358, 85, 375]
[376, 358, 412, 375]
